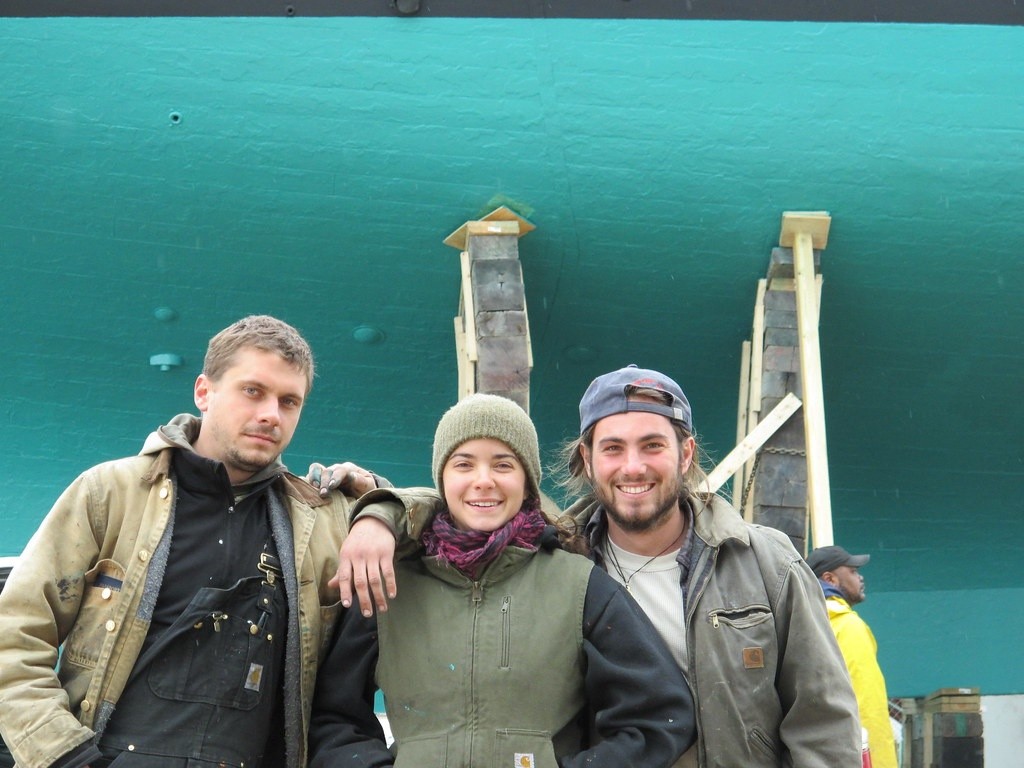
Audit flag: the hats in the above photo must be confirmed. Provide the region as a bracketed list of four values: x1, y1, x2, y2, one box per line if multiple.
[431, 394, 542, 506]
[804, 545, 871, 579]
[569, 364, 692, 477]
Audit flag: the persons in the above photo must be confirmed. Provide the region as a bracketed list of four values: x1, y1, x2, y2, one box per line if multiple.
[312, 392, 695, 768]
[298, 362, 862, 768]
[1, 314, 444, 768]
[804, 546, 899, 768]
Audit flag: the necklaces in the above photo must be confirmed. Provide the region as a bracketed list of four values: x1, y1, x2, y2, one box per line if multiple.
[606, 513, 686, 590]
